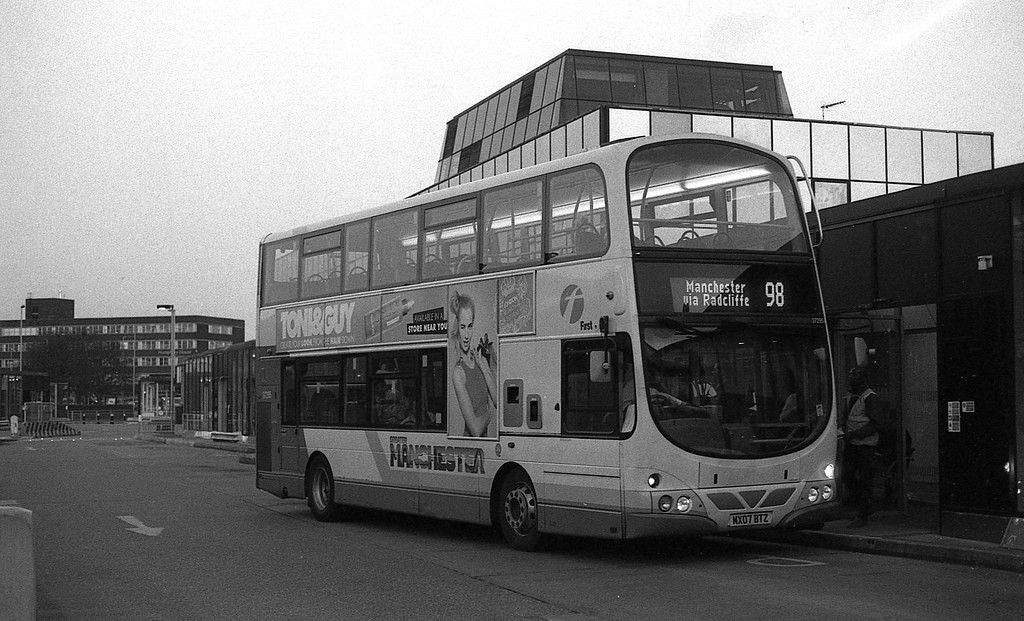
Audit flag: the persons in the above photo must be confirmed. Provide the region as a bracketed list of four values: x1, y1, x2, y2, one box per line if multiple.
[11, 413, 18, 436]
[780, 393, 801, 423]
[844, 368, 882, 528]
[401, 399, 435, 425]
[450, 291, 497, 437]
[623, 380, 686, 412]
[375, 380, 409, 424]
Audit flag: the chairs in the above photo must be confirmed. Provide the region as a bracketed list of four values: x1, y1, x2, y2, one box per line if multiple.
[269, 223, 734, 304]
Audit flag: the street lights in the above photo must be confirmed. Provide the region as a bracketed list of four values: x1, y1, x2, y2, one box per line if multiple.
[157, 304, 174, 435]
[20, 304, 26, 372]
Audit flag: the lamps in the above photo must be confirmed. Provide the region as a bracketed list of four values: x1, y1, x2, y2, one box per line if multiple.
[979, 256, 993, 270]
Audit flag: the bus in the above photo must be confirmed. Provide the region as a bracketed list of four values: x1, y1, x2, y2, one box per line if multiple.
[254, 132, 878, 551]
